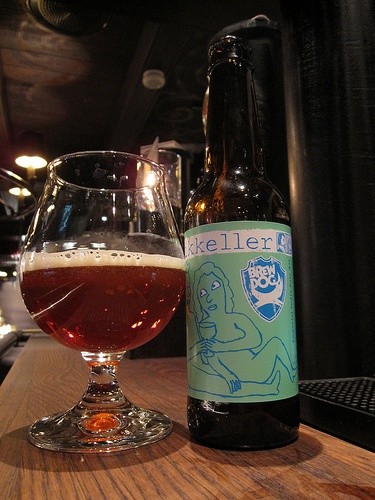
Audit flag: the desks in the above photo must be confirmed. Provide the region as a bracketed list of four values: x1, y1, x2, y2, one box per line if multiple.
[0, 333, 375, 500]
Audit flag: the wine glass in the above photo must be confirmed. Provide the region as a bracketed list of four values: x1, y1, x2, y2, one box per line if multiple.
[18, 150, 186, 453]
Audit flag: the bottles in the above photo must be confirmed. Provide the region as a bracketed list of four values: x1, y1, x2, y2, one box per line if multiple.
[186, 34, 302, 450]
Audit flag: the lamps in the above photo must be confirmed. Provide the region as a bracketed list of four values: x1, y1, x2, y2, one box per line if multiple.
[14, 129, 48, 170]
[8, 171, 31, 197]
[143, 69, 168, 91]
